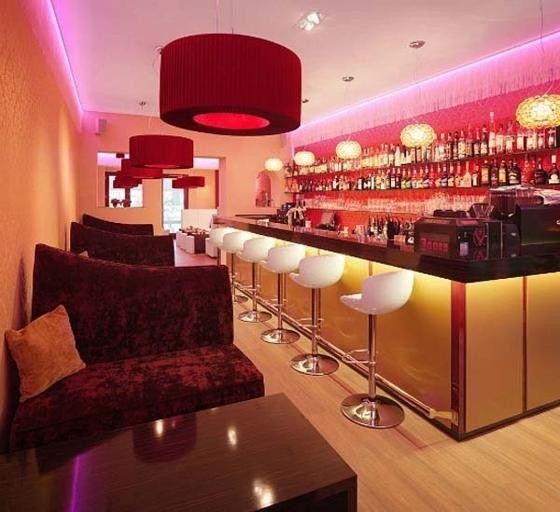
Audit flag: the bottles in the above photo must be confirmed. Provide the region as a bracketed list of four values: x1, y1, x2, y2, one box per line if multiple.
[400, 166, 408, 190]
[277, 201, 308, 229]
[442, 164, 450, 189]
[283, 165, 393, 193]
[499, 156, 522, 187]
[454, 160, 463, 188]
[496, 123, 504, 155]
[433, 133, 440, 162]
[479, 160, 492, 187]
[396, 167, 402, 190]
[457, 129, 465, 160]
[448, 163, 457, 188]
[422, 163, 431, 189]
[412, 166, 419, 190]
[466, 124, 473, 158]
[545, 126, 557, 150]
[489, 157, 499, 187]
[406, 165, 412, 189]
[533, 156, 548, 186]
[283, 130, 433, 180]
[370, 212, 414, 238]
[480, 126, 489, 158]
[525, 126, 536, 153]
[473, 128, 481, 157]
[472, 165, 481, 188]
[438, 134, 447, 162]
[453, 131, 458, 161]
[487, 111, 497, 157]
[445, 132, 454, 162]
[520, 154, 537, 185]
[516, 124, 526, 154]
[462, 162, 474, 188]
[537, 128, 545, 151]
[429, 165, 438, 189]
[547, 155, 560, 185]
[504, 120, 516, 154]
[432, 161, 443, 189]
[417, 167, 426, 189]
[390, 166, 396, 191]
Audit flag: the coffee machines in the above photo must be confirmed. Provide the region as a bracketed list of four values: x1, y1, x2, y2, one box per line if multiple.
[491, 192, 520, 259]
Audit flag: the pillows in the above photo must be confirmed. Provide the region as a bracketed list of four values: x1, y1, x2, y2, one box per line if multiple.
[4, 305, 87, 404]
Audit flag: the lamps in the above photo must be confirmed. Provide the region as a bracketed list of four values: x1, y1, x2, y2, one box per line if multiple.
[172, 170, 206, 190]
[121, 108, 163, 179]
[293, 145, 315, 167]
[156, 0, 302, 137]
[264, 156, 283, 172]
[336, 134, 362, 161]
[128, 101, 195, 170]
[514, 83, 560, 130]
[399, 118, 436, 149]
[112, 171, 142, 190]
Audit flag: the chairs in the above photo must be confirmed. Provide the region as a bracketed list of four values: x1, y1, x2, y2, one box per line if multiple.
[337, 269, 414, 429]
[261, 242, 305, 345]
[289, 252, 347, 377]
[235, 236, 277, 324]
[210, 226, 236, 266]
[222, 230, 258, 304]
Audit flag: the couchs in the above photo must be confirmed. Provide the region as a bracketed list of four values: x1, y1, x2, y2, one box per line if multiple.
[4, 243, 266, 450]
[80, 214, 155, 234]
[70, 223, 176, 268]
